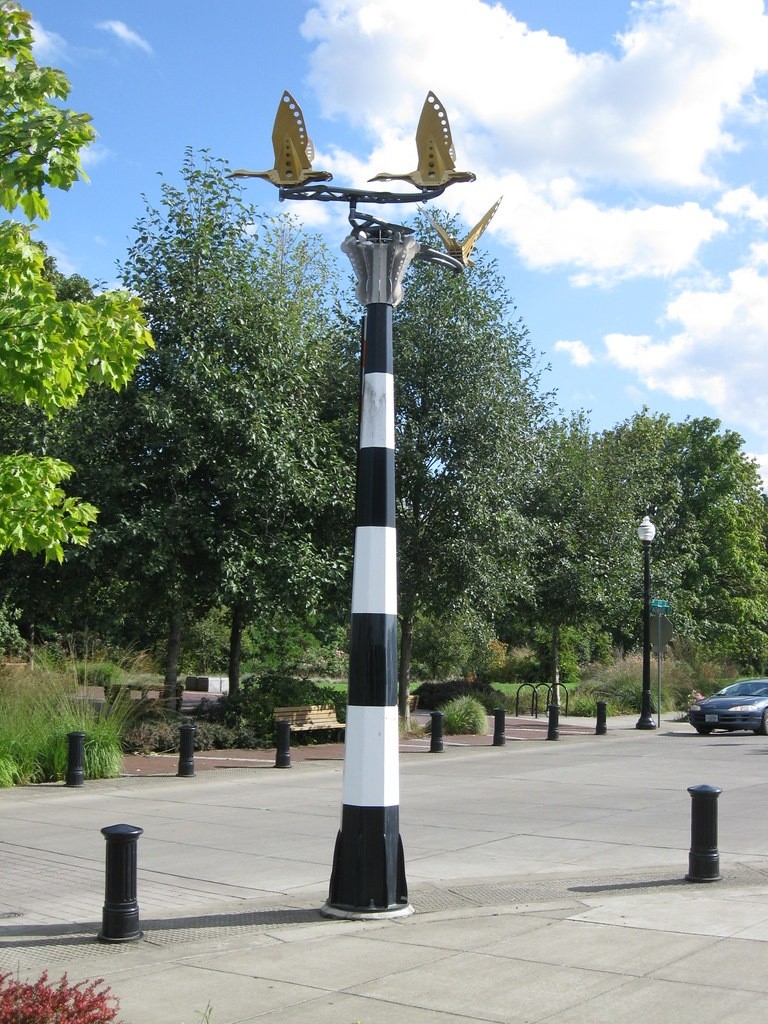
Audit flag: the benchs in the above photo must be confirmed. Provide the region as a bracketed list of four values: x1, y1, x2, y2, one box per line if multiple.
[273, 704, 347, 744]
[104, 684, 184, 712]
[398, 696, 419, 716]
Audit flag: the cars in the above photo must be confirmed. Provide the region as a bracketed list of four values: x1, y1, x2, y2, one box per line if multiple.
[686, 678, 768, 735]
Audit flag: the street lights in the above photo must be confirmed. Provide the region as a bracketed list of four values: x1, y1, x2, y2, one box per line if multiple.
[636, 515, 658, 730]
